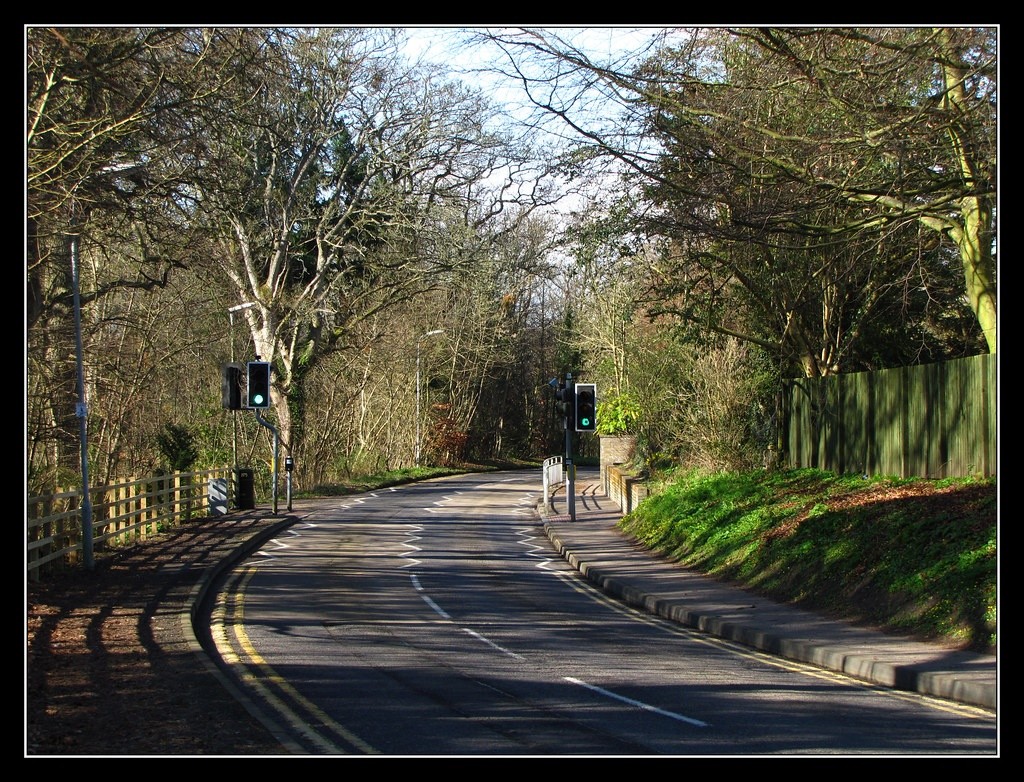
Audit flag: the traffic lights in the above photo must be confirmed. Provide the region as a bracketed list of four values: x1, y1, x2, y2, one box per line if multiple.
[575, 383, 598, 432]
[247, 362, 269, 408]
[553, 385, 568, 419]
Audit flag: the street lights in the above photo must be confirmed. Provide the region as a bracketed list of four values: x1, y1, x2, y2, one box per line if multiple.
[417, 329, 444, 468]
[228, 301, 258, 513]
[68, 156, 145, 577]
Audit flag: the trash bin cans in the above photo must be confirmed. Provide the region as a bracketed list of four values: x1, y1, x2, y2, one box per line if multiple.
[239, 468, 254, 509]
